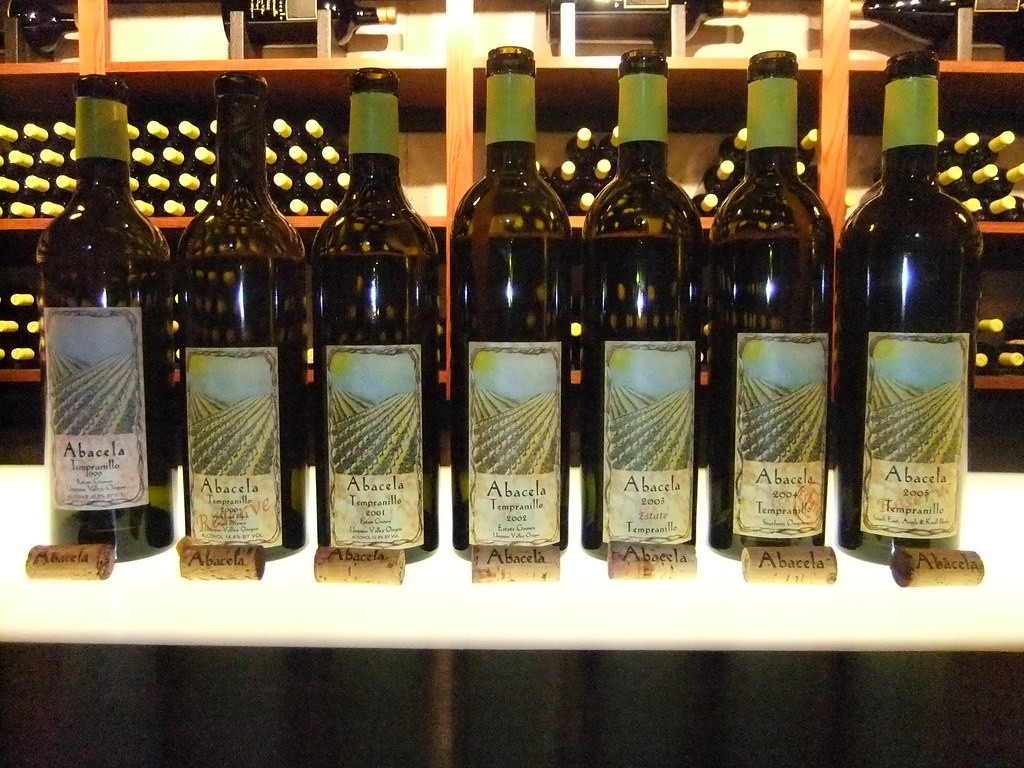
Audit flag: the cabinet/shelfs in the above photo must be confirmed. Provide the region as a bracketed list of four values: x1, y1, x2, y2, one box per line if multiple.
[0, 0, 1024, 400]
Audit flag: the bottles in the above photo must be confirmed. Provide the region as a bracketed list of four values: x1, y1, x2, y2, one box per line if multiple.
[0, 46, 1024, 566]
[546, 0, 750, 45]
[851, 0, 1024, 47]
[0, 0, 78, 61]
[221, 0, 397, 48]
